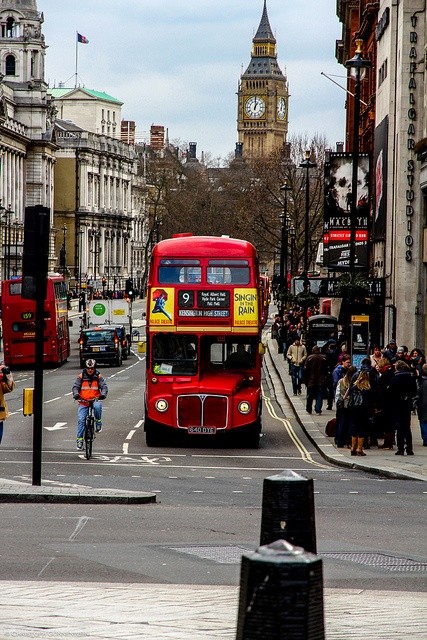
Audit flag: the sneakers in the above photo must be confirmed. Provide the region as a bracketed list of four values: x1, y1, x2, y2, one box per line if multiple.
[76, 438, 84, 450]
[95, 424, 102, 431]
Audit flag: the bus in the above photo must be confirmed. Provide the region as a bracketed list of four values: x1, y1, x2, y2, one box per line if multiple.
[2, 275, 71, 364]
[259, 277, 269, 327]
[144, 235, 262, 448]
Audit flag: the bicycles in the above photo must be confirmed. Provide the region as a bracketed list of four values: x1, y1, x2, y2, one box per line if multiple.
[74, 396, 105, 459]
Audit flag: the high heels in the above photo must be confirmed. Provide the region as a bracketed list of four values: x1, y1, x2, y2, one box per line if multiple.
[356, 447, 367, 456]
[350, 447, 358, 457]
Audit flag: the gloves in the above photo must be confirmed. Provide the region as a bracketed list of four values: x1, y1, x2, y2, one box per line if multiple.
[99, 396, 105, 399]
[73, 394, 80, 399]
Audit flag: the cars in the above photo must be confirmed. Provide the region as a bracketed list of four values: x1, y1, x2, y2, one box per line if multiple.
[97, 326, 128, 359]
[79, 330, 122, 366]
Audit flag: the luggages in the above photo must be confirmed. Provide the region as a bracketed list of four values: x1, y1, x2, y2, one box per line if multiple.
[324, 419, 338, 437]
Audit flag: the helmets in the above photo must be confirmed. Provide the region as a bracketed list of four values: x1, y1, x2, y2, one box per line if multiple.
[86, 359, 96, 368]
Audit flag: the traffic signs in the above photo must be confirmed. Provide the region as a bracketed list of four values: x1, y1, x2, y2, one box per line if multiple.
[94, 304, 105, 316]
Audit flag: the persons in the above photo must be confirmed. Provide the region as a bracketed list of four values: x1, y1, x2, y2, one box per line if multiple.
[72, 360, 109, 450]
[0, 364, 15, 446]
[81, 290, 137, 302]
[274, 303, 427, 455]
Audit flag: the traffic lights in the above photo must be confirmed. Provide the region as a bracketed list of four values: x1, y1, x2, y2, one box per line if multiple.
[102, 278, 106, 286]
[128, 280, 133, 295]
[22, 206, 49, 299]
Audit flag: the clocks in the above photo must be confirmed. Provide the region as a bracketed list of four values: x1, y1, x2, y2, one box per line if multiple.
[277, 97, 285, 121]
[244, 95, 265, 119]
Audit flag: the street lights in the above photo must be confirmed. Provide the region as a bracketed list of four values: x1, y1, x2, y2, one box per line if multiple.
[0, 203, 12, 275]
[281, 209, 298, 275]
[14, 219, 19, 272]
[345, 38, 370, 273]
[299, 156, 316, 277]
[279, 177, 292, 277]
